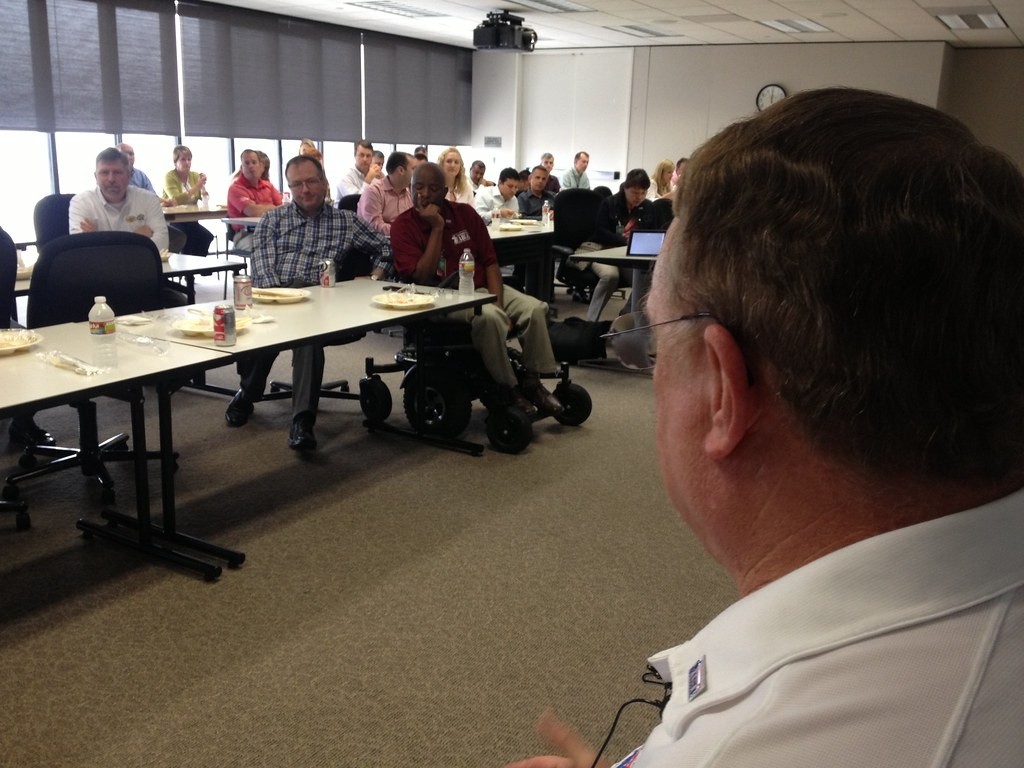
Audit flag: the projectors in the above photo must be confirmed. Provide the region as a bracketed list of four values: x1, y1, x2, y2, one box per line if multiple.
[473, 25, 538, 53]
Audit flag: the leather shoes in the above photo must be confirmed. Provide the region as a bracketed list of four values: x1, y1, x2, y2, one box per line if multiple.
[507, 389, 538, 415]
[523, 377, 565, 416]
[288, 414, 317, 450]
[225, 389, 254, 426]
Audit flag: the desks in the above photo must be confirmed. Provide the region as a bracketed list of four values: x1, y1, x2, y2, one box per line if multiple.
[1, 208, 657, 582]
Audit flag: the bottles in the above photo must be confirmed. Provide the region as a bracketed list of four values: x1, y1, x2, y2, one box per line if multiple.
[542, 200, 551, 226]
[282, 192, 290, 205]
[458, 249, 475, 296]
[201, 188, 209, 210]
[88, 296, 116, 372]
[491, 205, 501, 231]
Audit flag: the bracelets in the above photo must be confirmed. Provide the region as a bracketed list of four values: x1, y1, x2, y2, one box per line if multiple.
[370, 274, 382, 280]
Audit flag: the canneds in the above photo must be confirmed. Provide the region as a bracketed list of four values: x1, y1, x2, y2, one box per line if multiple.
[233, 275, 252, 310]
[213, 305, 236, 346]
[318, 258, 335, 287]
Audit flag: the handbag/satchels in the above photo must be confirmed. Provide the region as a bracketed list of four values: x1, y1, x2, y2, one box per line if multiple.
[548, 315, 613, 360]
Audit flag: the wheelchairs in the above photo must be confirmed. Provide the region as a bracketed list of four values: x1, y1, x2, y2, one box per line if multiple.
[358, 253, 593, 454]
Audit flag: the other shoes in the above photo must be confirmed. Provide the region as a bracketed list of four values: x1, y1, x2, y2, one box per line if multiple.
[8, 421, 56, 445]
[573, 290, 589, 304]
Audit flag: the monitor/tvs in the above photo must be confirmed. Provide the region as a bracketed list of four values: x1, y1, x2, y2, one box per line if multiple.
[627, 229, 667, 257]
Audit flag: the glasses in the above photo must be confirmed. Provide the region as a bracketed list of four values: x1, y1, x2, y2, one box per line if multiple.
[599, 310, 755, 388]
[289, 174, 322, 190]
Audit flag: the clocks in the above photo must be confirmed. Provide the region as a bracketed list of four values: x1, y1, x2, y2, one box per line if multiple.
[755, 83, 787, 112]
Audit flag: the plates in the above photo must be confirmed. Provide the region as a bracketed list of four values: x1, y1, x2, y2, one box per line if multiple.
[159, 252, 171, 261]
[499, 225, 523, 231]
[509, 218, 537, 224]
[371, 293, 434, 309]
[0, 332, 44, 355]
[16, 266, 31, 279]
[171, 317, 252, 338]
[252, 288, 312, 303]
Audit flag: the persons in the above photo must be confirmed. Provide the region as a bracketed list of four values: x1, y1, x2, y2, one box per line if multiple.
[116, 144, 186, 253]
[389, 162, 563, 417]
[501, 87, 1024, 768]
[335, 139, 691, 323]
[298, 139, 333, 206]
[163, 146, 214, 256]
[227, 150, 282, 252]
[224, 156, 392, 451]
[68, 147, 186, 306]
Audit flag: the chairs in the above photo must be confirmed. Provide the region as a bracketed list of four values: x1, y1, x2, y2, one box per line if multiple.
[35, 194, 76, 254]
[5, 230, 195, 501]
[551, 189, 627, 299]
[0, 232, 32, 528]
[239, 242, 372, 410]
